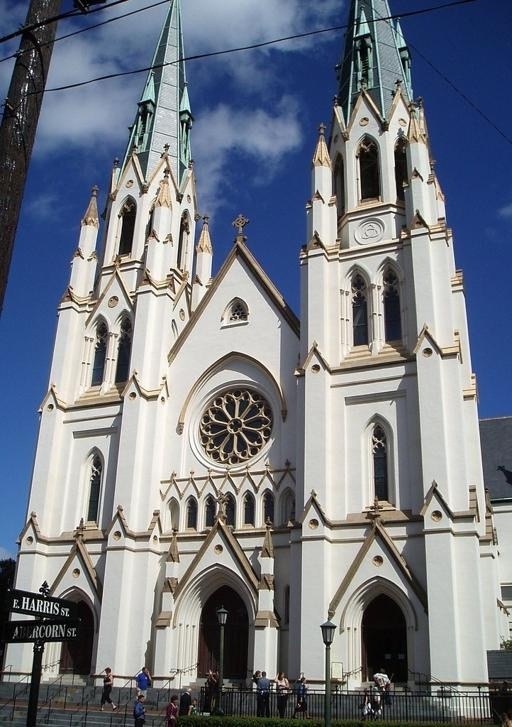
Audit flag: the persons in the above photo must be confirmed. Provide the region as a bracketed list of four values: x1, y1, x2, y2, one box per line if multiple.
[133, 693, 146, 726]
[165, 667, 392, 726]
[136, 665, 153, 712]
[99, 666, 118, 711]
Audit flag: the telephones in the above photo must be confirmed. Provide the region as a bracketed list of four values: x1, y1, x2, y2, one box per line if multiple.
[1, 617, 84, 644]
[5, 588, 78, 622]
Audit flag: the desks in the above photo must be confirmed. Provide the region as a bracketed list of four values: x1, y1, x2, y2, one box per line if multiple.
[319, 618, 337, 726]
[215, 602, 230, 717]
